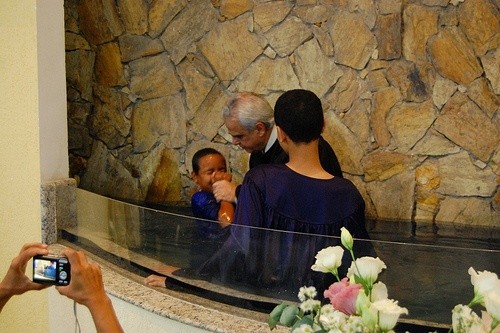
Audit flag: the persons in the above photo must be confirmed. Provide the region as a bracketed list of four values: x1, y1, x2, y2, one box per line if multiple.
[211, 95, 344, 205]
[144, 90, 385, 314]
[1, 243, 126, 333]
[191, 147, 234, 248]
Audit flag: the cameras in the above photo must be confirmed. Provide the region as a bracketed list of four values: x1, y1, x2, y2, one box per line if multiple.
[32, 254, 71, 286]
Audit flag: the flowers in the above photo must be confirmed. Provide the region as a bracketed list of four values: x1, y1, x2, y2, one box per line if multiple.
[447, 266, 500, 333]
[267, 227, 408, 333]
